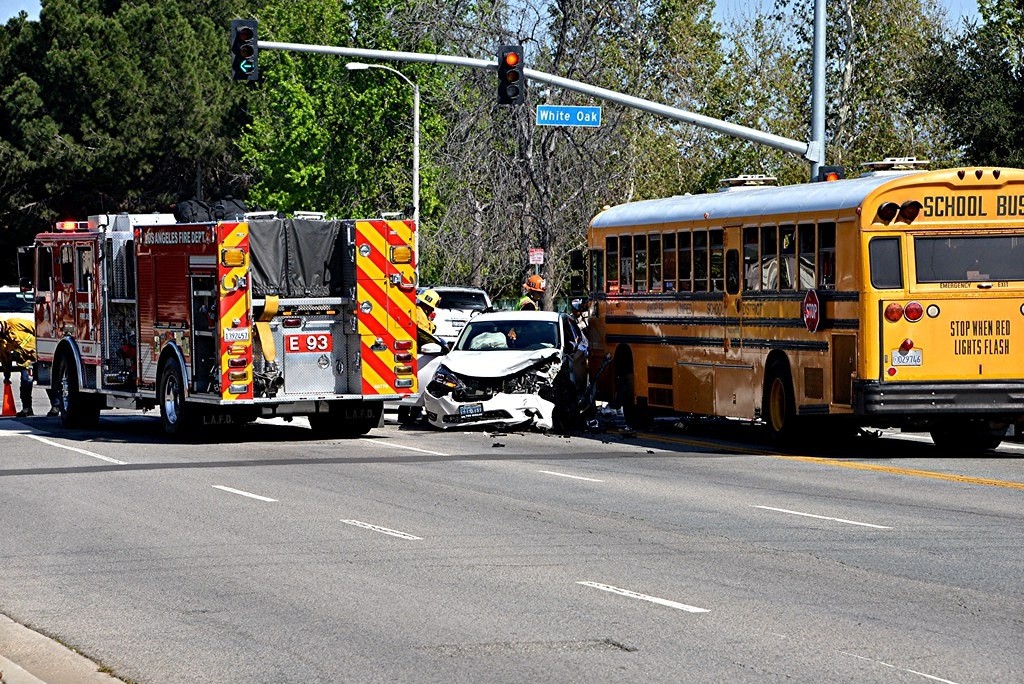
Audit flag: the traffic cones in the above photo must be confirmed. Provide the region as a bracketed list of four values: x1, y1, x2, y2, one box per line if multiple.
[0, 384, 18, 418]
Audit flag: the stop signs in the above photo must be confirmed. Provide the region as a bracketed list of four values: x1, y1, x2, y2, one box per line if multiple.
[803, 290, 820, 333]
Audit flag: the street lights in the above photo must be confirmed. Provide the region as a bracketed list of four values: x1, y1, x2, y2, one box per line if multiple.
[345, 63, 420, 288]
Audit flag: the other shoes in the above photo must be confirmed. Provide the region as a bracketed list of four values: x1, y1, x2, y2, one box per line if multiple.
[48, 407, 61, 415]
[17, 406, 33, 417]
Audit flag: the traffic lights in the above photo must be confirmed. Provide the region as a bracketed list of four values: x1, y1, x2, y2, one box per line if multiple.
[497, 46, 525, 105]
[230, 20, 258, 80]
[818, 164, 845, 182]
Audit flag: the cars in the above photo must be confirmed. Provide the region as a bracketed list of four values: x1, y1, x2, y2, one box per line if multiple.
[0, 285, 36, 369]
[404, 286, 594, 434]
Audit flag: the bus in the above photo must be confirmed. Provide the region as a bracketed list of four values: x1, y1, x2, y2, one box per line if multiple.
[570, 156, 1024, 456]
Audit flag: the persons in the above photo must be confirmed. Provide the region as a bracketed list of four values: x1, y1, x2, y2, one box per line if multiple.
[398, 291, 439, 425]
[570, 299, 588, 342]
[20, 279, 32, 291]
[0, 317, 60, 417]
[509, 275, 546, 349]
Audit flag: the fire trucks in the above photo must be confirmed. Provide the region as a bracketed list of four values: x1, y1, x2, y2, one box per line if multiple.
[17, 212, 420, 441]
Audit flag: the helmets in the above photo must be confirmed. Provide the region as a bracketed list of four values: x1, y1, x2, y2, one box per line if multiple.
[416, 289, 441, 308]
[523, 275, 547, 292]
